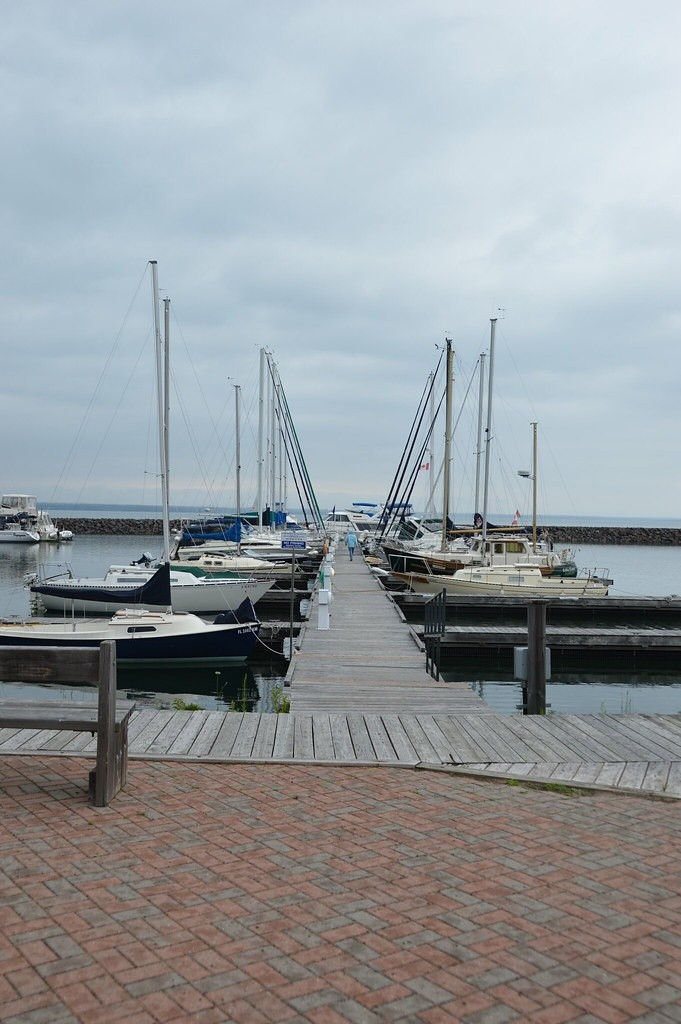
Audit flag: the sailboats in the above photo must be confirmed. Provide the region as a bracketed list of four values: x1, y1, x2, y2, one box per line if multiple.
[101, 257, 331, 601]
[370, 318, 579, 578]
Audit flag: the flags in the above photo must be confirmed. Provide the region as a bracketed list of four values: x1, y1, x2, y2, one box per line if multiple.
[420, 463, 429, 470]
[512, 510, 520, 524]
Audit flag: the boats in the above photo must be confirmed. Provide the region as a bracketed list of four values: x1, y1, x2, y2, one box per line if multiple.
[36, 562, 277, 616]
[37, 510, 75, 542]
[389, 565, 615, 597]
[352, 503, 444, 533]
[309, 503, 373, 533]
[0, 494, 41, 543]
[0, 608, 261, 668]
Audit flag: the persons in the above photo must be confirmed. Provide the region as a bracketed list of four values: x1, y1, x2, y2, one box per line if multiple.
[345, 530, 358, 561]
